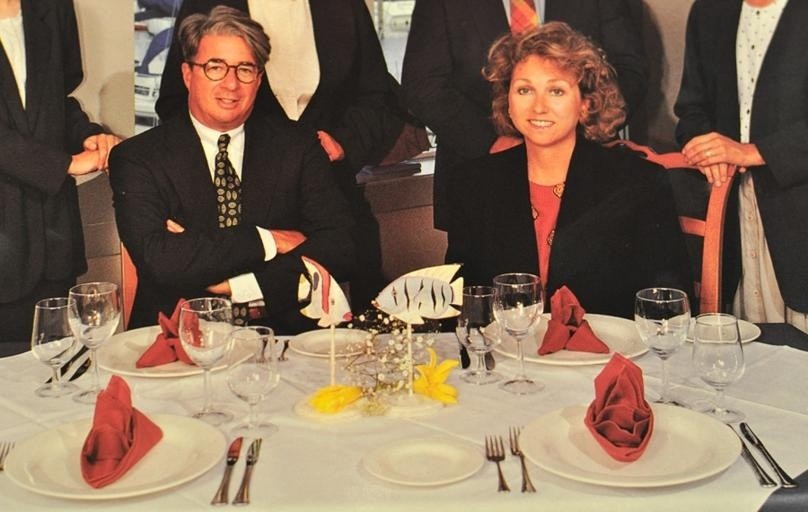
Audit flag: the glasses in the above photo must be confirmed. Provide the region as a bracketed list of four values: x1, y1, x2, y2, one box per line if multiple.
[188, 58, 262, 85]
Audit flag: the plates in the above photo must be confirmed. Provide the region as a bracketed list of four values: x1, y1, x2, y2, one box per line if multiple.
[515, 400, 744, 490]
[483, 310, 651, 366]
[2, 410, 229, 501]
[287, 329, 373, 359]
[97, 322, 262, 376]
[680, 314, 762, 347]
[361, 435, 486, 488]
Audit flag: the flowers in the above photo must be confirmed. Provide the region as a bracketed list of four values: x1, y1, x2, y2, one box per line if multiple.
[406, 342, 462, 409]
[304, 379, 365, 418]
[326, 307, 418, 415]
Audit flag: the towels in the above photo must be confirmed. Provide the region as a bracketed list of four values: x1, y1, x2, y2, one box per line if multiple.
[584, 345, 660, 467]
[77, 371, 164, 491]
[133, 298, 210, 368]
[535, 280, 614, 359]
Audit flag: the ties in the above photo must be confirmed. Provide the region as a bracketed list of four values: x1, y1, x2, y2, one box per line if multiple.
[213, 135, 248, 326]
[510, 0, 540, 34]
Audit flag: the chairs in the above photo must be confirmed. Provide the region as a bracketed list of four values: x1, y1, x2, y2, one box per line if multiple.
[119, 230, 139, 329]
[616, 135, 742, 324]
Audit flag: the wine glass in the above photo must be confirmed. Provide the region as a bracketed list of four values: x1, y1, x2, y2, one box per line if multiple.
[32, 298, 78, 400]
[691, 312, 744, 423]
[633, 287, 691, 408]
[176, 299, 235, 425]
[68, 282, 122, 405]
[223, 325, 280, 439]
[450, 283, 501, 387]
[491, 270, 545, 398]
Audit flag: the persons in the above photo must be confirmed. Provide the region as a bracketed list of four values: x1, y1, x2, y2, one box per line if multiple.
[396, 0, 649, 257]
[674, 0, 808, 354]
[1, 0, 127, 357]
[152, 1, 391, 175]
[437, 18, 697, 332]
[108, 5, 359, 337]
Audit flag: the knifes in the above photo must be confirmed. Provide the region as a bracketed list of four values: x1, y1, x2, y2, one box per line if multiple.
[725, 422, 778, 488]
[231, 437, 262, 505]
[41, 344, 92, 387]
[448, 327, 494, 371]
[740, 421, 799, 489]
[211, 436, 242, 505]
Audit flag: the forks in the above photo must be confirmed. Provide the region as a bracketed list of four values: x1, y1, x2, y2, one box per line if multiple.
[483, 435, 511, 494]
[508, 421, 538, 495]
[0, 439, 14, 471]
